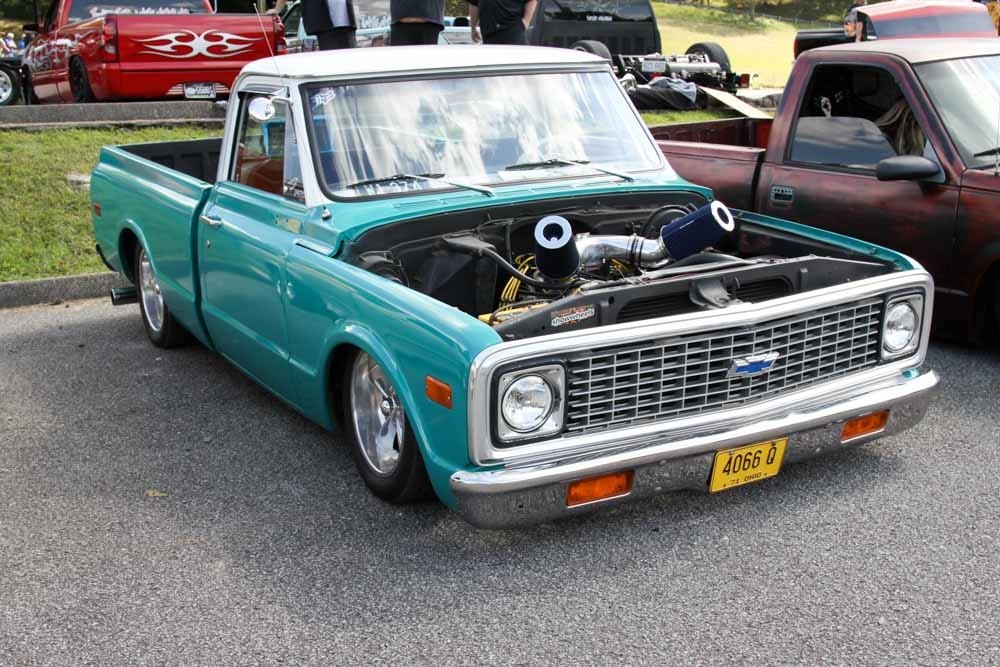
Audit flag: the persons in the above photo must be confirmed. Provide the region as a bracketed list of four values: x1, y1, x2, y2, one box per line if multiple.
[470, 0, 537, 45]
[267, 0, 356, 50]
[391, 0, 445, 46]
[0, 32, 26, 58]
[857, 90, 924, 158]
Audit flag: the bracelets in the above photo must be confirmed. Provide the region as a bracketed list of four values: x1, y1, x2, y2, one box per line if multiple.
[472, 30, 476, 32]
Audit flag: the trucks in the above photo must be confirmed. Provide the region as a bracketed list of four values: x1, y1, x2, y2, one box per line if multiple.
[522, 0, 750, 93]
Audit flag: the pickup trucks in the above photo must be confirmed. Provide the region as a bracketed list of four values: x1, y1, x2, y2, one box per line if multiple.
[650, 0, 1000, 355]
[0, 0, 483, 104]
[89, 44, 941, 531]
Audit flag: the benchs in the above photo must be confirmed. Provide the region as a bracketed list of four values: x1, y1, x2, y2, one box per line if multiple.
[245, 144, 479, 195]
